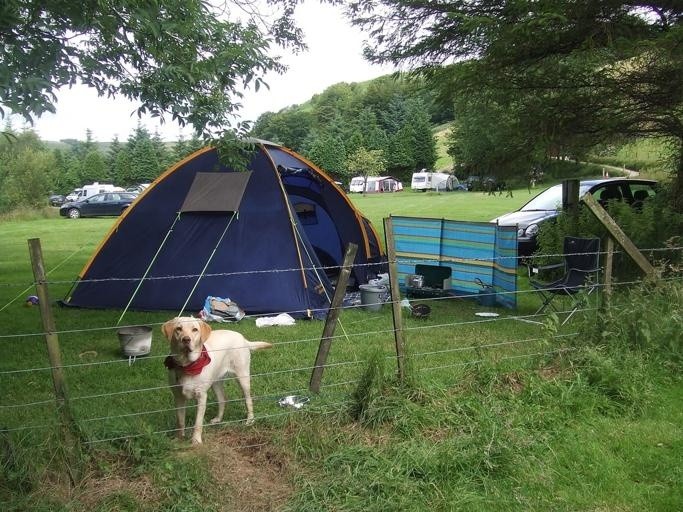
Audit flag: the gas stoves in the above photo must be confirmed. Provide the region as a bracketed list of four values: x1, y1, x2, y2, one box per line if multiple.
[406, 285, 450, 296]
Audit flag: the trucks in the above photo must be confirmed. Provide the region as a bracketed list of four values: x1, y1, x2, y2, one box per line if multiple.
[411, 173, 453, 192]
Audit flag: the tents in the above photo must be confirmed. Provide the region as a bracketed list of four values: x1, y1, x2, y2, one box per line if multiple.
[57, 137, 384, 320]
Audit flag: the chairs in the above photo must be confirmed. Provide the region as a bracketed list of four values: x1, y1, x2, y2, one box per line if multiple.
[527, 236, 602, 327]
[600, 190, 621, 207]
[634, 190, 649, 201]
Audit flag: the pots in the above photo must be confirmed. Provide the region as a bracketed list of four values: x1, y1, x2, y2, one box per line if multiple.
[408, 275, 424, 288]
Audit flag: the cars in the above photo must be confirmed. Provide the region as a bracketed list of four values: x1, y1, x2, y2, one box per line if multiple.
[60, 193, 135, 219]
[490, 179, 662, 265]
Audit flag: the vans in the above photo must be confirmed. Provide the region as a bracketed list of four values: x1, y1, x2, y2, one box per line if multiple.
[51, 182, 113, 206]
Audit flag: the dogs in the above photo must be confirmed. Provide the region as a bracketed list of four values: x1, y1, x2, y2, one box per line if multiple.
[160, 314, 273, 446]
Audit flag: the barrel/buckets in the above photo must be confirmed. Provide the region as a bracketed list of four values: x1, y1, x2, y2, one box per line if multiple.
[359, 283, 386, 311]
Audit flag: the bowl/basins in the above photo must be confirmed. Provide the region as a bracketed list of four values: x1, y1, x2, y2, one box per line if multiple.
[280, 395, 310, 408]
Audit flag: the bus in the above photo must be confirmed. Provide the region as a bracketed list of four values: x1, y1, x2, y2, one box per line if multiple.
[349, 177, 371, 193]
